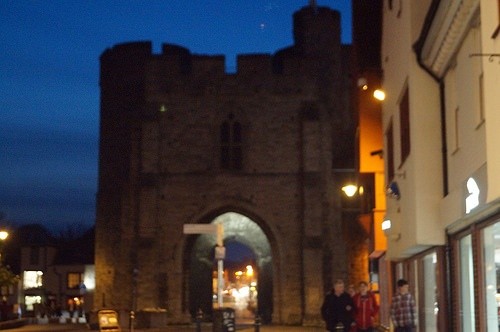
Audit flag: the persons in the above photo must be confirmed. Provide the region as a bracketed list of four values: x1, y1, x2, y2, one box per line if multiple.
[389, 279, 417, 332]
[350, 279, 381, 331]
[320, 279, 355, 332]
